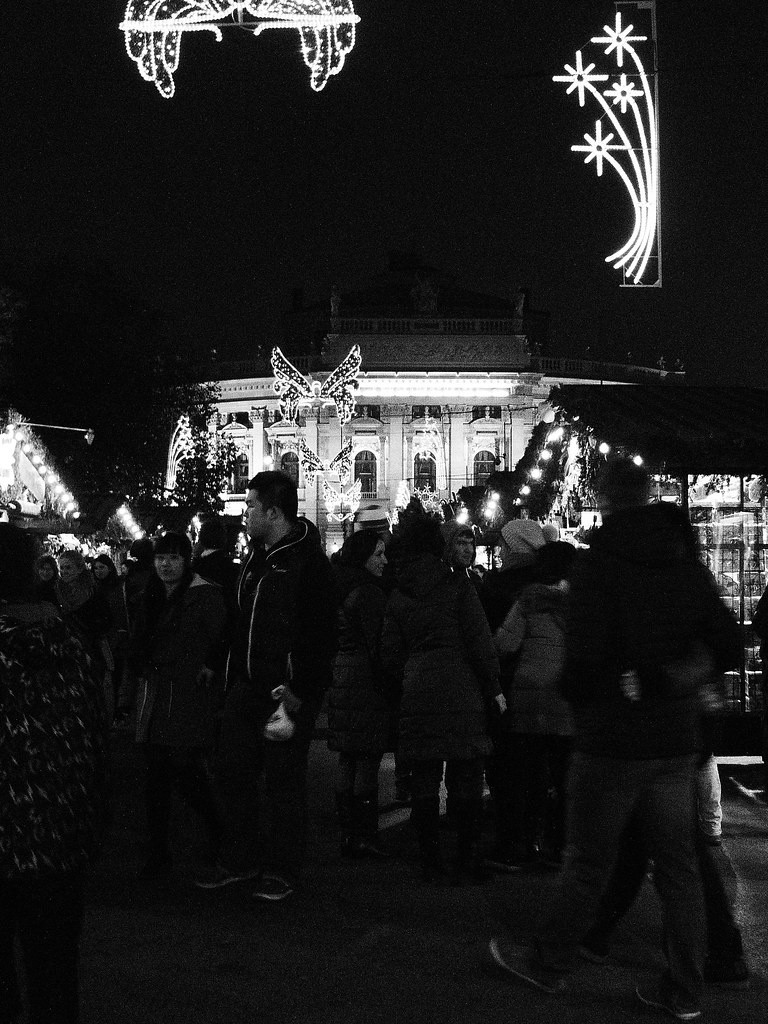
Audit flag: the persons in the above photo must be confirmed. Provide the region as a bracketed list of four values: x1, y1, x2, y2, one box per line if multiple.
[329, 285, 340, 316]
[486, 455, 768, 1019]
[513, 284, 524, 317]
[0, 472, 575, 1023]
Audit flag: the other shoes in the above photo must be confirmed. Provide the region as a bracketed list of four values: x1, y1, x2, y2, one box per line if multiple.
[196, 862, 258, 889]
[480, 847, 523, 873]
[252, 879, 291, 901]
[490, 938, 564, 993]
[536, 845, 564, 868]
[636, 983, 702, 1020]
[551, 922, 613, 963]
[696, 955, 748, 989]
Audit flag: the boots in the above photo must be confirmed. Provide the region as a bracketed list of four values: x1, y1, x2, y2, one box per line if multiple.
[339, 787, 404, 861]
